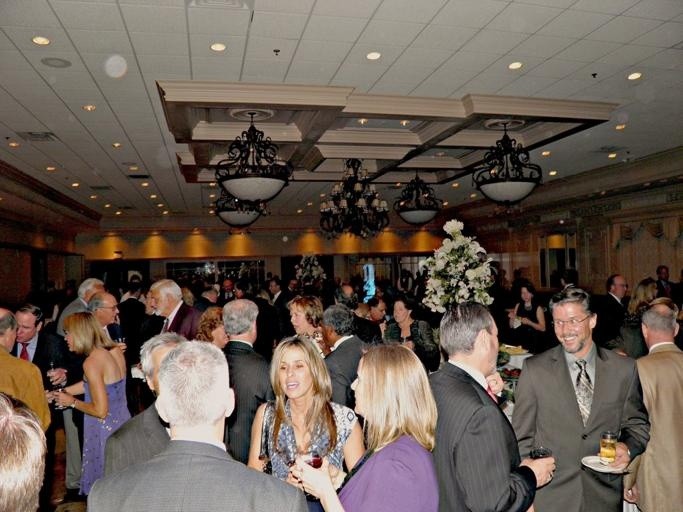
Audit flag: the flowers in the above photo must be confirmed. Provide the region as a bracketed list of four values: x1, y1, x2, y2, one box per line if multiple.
[294, 250, 327, 302]
[419, 219, 498, 313]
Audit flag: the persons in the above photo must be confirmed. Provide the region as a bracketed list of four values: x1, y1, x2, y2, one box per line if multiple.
[247, 338, 367, 504]
[85, 342, 309, 512]
[0, 395, 47, 511]
[287, 342, 438, 512]
[623, 298, 681, 512]
[428, 302, 555, 512]
[0, 262, 272, 494]
[268, 263, 683, 357]
[512, 287, 651, 511]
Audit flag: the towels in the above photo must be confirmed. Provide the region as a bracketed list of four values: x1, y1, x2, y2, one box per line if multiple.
[131, 366, 147, 383]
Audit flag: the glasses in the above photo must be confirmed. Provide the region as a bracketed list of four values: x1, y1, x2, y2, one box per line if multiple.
[615, 284, 629, 289]
[99, 305, 119, 311]
[552, 315, 591, 326]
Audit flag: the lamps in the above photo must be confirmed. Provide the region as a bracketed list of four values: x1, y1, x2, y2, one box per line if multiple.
[472, 120, 544, 207]
[214, 109, 294, 204]
[318, 157, 390, 242]
[113, 251, 123, 261]
[393, 170, 443, 227]
[213, 189, 271, 235]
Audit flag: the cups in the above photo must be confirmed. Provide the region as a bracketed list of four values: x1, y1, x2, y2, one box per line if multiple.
[113, 337, 125, 343]
[598, 431, 617, 464]
[529, 446, 552, 459]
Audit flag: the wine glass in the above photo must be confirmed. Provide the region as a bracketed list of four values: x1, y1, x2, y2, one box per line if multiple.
[303, 446, 323, 501]
[53, 387, 68, 412]
[279, 445, 310, 495]
[48, 362, 59, 390]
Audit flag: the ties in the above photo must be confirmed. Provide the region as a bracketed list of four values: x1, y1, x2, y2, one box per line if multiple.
[574, 359, 593, 426]
[161, 318, 170, 333]
[664, 282, 671, 297]
[227, 292, 231, 300]
[20, 342, 30, 361]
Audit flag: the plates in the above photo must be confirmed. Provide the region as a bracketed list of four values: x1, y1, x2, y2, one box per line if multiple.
[582, 454, 626, 473]
